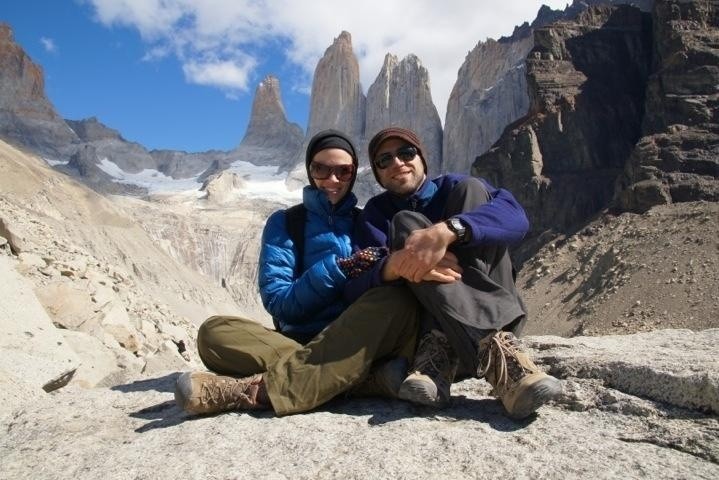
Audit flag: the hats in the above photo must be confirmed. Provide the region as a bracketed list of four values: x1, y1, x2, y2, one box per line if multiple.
[368, 127, 428, 188]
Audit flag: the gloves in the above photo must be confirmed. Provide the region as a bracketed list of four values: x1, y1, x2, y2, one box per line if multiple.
[336, 246, 387, 278]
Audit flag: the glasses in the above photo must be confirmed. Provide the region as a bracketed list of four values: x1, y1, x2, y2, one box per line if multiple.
[373, 144, 418, 169]
[308, 160, 355, 182]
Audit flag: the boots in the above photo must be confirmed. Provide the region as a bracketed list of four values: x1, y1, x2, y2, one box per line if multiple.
[341, 355, 406, 401]
[475, 330, 562, 421]
[175, 370, 264, 414]
[397, 328, 460, 409]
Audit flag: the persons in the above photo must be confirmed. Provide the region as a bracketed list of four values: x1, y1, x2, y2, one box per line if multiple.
[350, 123, 565, 423]
[174, 128, 422, 420]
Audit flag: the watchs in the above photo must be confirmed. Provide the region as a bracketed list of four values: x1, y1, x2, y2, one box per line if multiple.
[441, 217, 468, 247]
